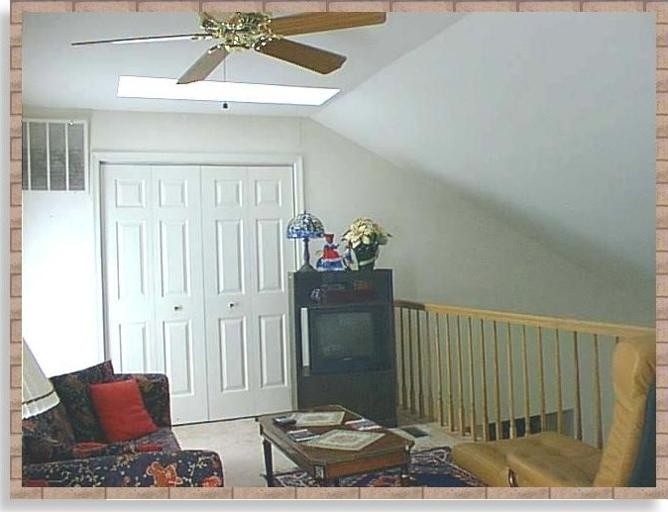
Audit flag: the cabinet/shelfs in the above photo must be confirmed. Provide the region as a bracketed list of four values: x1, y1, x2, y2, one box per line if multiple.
[292, 271, 399, 430]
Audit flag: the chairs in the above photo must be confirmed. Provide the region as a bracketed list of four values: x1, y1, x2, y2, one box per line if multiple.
[452, 335, 656, 485]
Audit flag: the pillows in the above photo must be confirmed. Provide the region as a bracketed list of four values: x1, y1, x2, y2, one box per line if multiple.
[23, 360, 164, 463]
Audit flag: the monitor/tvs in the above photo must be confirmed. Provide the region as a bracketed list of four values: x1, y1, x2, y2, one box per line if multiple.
[299, 302, 391, 377]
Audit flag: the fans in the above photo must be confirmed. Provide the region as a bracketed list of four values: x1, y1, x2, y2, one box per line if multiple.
[70, 11, 388, 109]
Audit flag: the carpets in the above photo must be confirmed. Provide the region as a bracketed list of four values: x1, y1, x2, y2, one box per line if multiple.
[259, 446, 483, 487]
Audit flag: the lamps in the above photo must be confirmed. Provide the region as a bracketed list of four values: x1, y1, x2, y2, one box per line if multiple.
[20, 338, 60, 423]
[287, 209, 325, 272]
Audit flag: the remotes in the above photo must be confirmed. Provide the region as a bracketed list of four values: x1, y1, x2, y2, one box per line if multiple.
[272, 416, 296, 427]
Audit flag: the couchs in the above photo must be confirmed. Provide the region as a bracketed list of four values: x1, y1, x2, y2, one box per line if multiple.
[22, 373, 224, 486]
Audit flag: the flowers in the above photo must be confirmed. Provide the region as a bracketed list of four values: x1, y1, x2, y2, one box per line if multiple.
[340, 216, 394, 252]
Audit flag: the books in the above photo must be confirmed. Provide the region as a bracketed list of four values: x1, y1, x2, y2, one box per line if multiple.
[289, 408, 386, 452]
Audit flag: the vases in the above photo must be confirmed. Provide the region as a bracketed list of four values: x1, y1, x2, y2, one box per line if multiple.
[353, 248, 378, 270]
